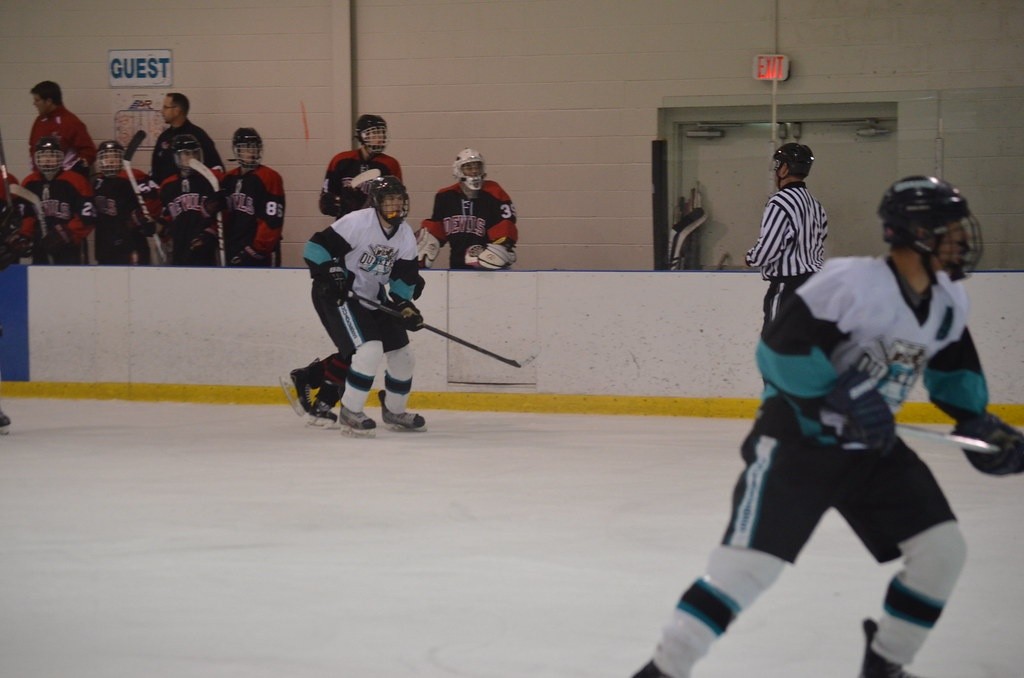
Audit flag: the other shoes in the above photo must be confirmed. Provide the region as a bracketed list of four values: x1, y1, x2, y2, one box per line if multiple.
[0, 408, 11, 435]
[859, 619, 913, 678]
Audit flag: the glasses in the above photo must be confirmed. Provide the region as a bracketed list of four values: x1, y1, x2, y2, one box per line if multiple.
[163, 105, 178, 110]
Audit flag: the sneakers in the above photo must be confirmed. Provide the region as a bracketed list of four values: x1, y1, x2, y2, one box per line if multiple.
[378, 390, 428, 432]
[279, 357, 320, 420]
[305, 402, 339, 429]
[339, 404, 376, 437]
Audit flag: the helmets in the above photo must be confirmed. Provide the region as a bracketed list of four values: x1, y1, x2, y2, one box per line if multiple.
[96, 141, 126, 178]
[354, 115, 389, 155]
[169, 134, 204, 173]
[231, 127, 263, 167]
[874, 175, 982, 280]
[33, 136, 64, 175]
[369, 175, 409, 225]
[773, 142, 814, 177]
[451, 147, 484, 185]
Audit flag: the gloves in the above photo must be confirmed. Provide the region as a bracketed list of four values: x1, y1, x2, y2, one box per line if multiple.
[5, 230, 33, 258]
[325, 264, 349, 294]
[37, 223, 71, 258]
[396, 299, 424, 331]
[227, 248, 251, 266]
[478, 243, 510, 271]
[183, 231, 216, 263]
[129, 208, 148, 231]
[951, 410, 1024, 477]
[415, 229, 440, 268]
[823, 368, 894, 456]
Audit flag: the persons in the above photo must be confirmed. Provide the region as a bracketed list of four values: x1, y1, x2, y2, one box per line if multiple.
[317, 115, 403, 222]
[745, 142, 829, 328]
[85, 140, 162, 267]
[203, 126, 286, 269]
[160, 133, 226, 268]
[0, 169, 17, 200]
[632, 176, 1024, 678]
[28, 79, 96, 177]
[19, 138, 94, 266]
[278, 257, 424, 429]
[303, 175, 427, 438]
[413, 148, 518, 271]
[150, 93, 226, 185]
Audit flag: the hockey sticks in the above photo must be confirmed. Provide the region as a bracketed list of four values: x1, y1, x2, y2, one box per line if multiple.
[188, 159, 225, 267]
[894, 423, 1002, 454]
[9, 182, 55, 264]
[345, 289, 542, 368]
[351, 168, 381, 189]
[0, 131, 12, 207]
[121, 130, 167, 263]
